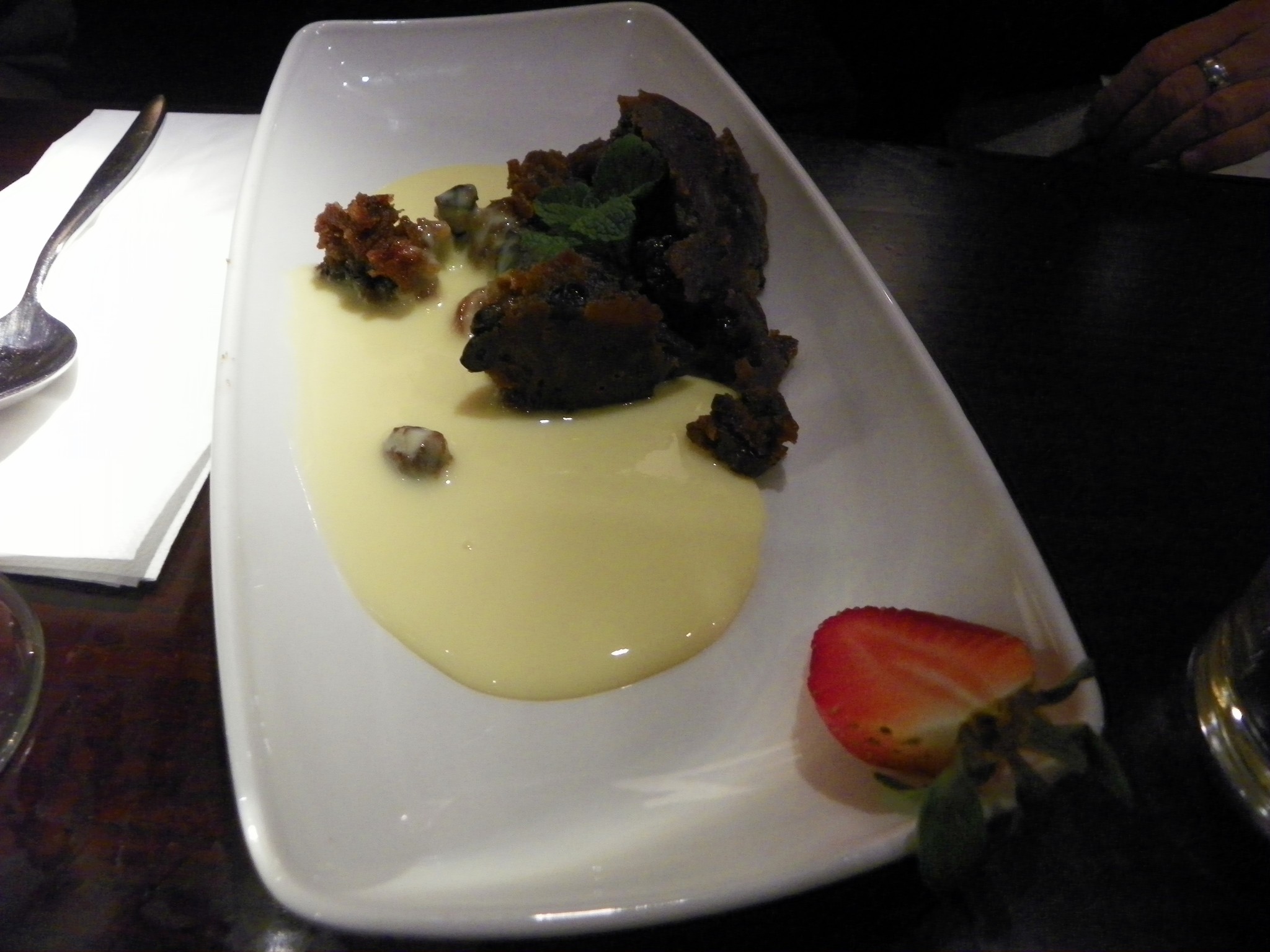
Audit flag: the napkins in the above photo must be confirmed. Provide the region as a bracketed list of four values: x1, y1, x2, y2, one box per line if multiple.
[1, 108, 261, 587]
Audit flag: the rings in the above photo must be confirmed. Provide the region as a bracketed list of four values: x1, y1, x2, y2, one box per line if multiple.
[1199, 51, 1231, 93]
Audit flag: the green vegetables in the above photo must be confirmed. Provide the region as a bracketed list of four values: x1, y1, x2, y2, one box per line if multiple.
[513, 132, 667, 269]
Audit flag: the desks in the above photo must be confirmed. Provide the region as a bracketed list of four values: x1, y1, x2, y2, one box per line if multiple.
[0, 97, 1270, 952]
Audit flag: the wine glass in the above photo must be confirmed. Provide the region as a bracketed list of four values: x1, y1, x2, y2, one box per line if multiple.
[1183, 589, 1269, 836]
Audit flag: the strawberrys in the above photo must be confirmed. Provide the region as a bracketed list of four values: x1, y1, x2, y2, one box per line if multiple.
[808, 607, 1128, 864]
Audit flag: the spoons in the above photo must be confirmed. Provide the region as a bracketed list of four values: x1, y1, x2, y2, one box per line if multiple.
[0, 92, 171, 412]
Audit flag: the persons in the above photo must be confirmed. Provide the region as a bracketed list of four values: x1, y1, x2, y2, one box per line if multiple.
[1078, 1, 1270, 172]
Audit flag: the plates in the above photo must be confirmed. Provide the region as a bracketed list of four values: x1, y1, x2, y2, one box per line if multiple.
[211, 0, 1109, 946]
